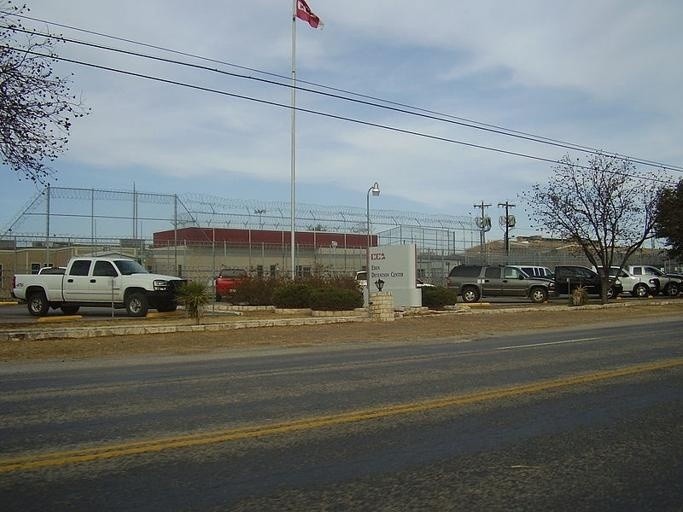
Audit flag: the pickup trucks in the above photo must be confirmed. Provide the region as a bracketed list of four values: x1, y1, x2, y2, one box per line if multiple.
[9, 255, 189, 318]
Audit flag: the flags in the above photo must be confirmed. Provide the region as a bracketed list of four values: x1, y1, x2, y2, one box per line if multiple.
[295, 0, 324, 31]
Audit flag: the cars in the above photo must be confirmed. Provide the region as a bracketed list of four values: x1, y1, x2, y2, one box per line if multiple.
[214, 268, 250, 304]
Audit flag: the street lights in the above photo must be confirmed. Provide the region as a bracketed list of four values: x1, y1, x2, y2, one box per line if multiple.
[366, 181, 381, 305]
